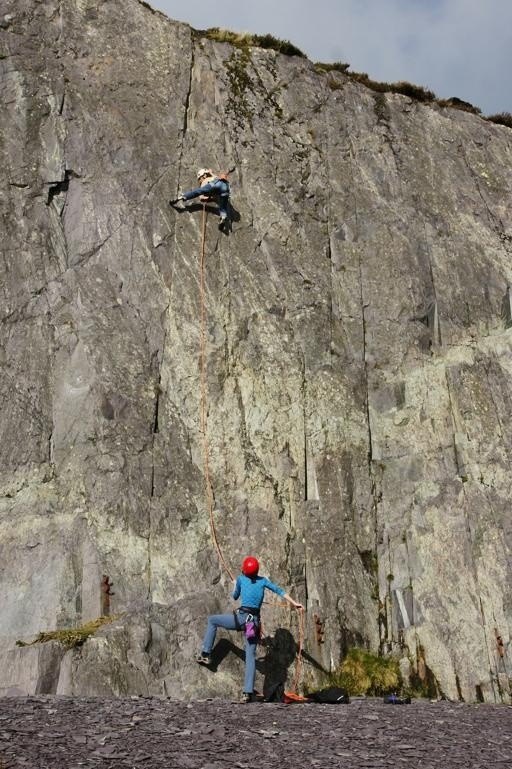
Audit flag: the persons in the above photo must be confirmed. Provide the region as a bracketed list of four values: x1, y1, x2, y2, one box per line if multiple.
[168, 167, 231, 232]
[194, 556, 305, 702]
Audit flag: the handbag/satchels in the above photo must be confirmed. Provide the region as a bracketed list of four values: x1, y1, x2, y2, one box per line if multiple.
[303, 686, 350, 704]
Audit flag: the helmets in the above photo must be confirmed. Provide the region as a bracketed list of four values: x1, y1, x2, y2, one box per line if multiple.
[241, 556, 259, 576]
[197, 167, 209, 180]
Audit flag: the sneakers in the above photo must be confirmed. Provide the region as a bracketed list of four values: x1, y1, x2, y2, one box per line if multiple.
[169, 197, 177, 205]
[242, 693, 250, 701]
[193, 652, 210, 664]
[218, 216, 229, 229]
